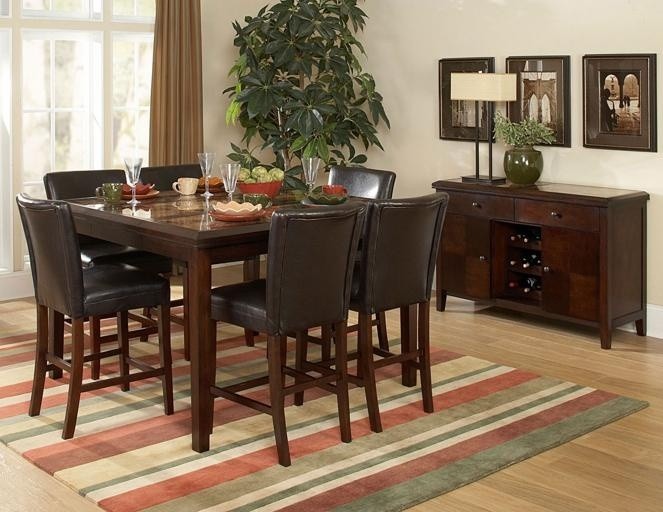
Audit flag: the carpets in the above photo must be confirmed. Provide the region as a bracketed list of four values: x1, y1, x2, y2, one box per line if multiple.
[0, 303, 650, 512]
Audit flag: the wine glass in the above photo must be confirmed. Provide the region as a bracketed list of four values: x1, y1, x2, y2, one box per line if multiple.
[301, 158, 320, 190]
[122, 157, 144, 204]
[219, 163, 242, 202]
[199, 196, 217, 232]
[196, 152, 216, 196]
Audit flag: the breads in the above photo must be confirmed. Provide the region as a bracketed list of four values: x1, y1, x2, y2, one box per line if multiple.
[198, 176, 223, 188]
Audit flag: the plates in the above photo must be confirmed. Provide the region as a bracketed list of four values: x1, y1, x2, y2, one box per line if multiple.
[208, 201, 265, 222]
[116, 183, 160, 200]
[197, 176, 224, 193]
[301, 193, 347, 208]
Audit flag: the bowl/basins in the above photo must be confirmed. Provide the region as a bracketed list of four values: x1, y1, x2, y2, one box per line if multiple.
[237, 179, 283, 200]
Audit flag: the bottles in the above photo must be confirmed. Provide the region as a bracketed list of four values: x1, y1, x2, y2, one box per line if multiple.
[507, 230, 542, 294]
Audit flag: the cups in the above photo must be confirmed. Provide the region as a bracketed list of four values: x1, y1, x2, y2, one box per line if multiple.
[322, 185, 344, 196]
[171, 194, 205, 211]
[172, 177, 199, 195]
[95, 182, 123, 203]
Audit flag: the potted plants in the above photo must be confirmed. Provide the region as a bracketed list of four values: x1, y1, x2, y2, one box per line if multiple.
[490, 110, 558, 185]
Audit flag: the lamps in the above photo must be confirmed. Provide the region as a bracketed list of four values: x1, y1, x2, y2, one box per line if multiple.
[449, 71, 517, 184]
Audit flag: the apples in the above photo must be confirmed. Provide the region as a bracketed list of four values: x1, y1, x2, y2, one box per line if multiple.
[238, 166, 285, 184]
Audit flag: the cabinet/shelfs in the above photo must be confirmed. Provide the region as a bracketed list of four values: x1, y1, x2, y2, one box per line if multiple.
[430, 175, 650, 349]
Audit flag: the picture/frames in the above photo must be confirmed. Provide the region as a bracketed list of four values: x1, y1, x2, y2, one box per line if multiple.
[437, 53, 658, 152]
